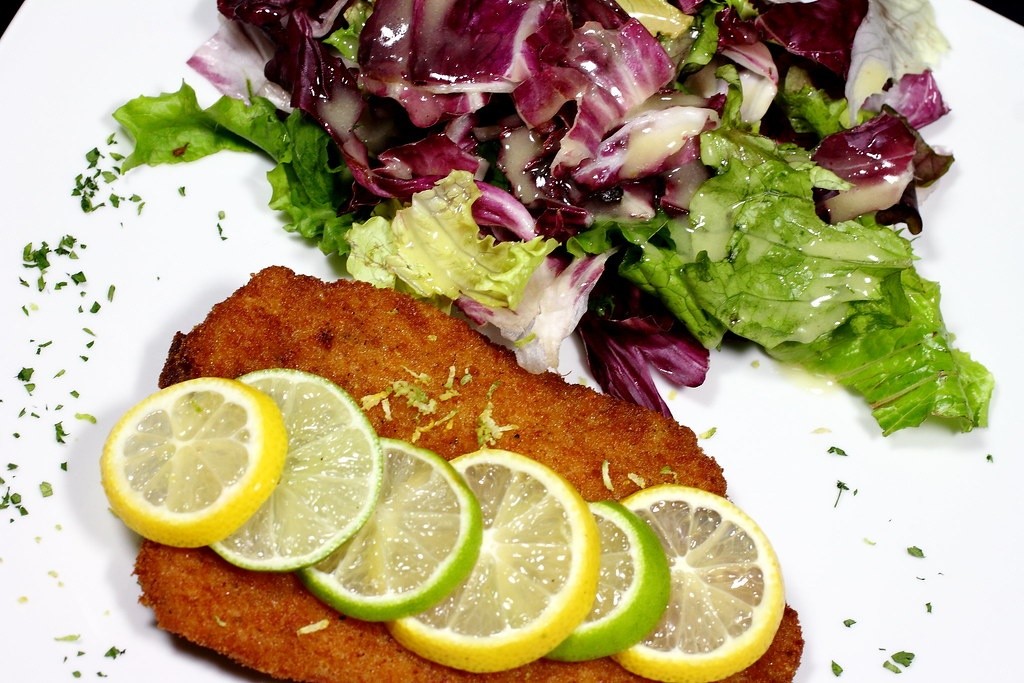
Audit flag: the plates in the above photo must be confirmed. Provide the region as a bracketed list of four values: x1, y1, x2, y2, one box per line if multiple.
[1, 0, 1024, 683]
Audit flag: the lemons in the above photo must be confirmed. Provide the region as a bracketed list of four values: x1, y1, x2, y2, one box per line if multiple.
[102, 369, 783, 681]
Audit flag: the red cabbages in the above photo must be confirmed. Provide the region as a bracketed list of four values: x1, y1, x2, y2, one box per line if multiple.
[186, 0, 948, 419]
